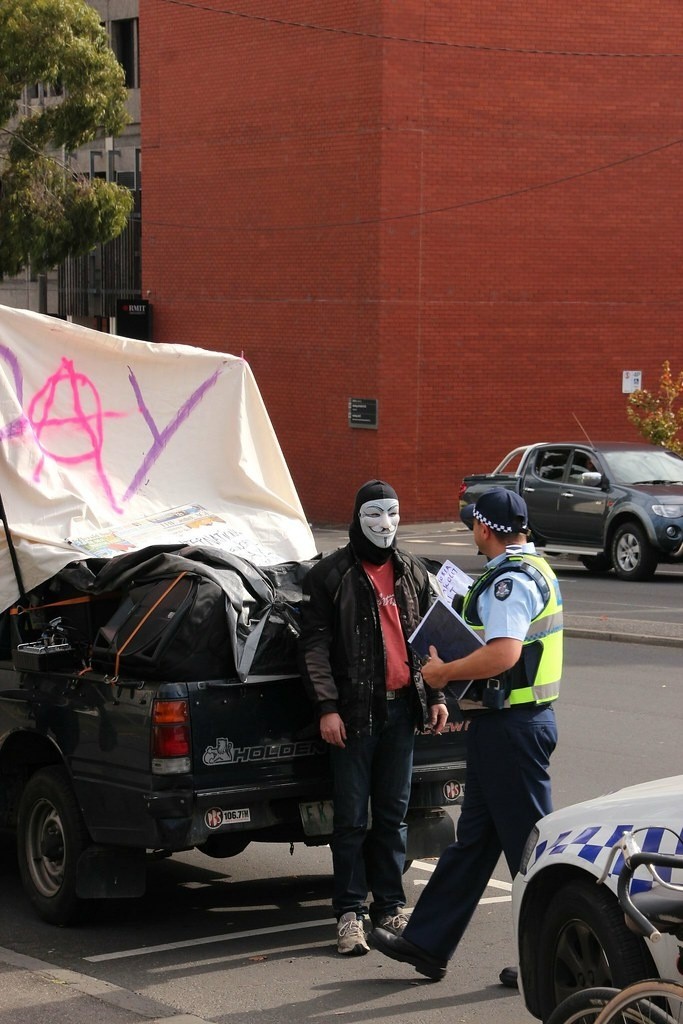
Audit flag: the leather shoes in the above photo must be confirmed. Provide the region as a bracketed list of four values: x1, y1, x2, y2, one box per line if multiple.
[499, 966, 517, 987]
[367, 927, 447, 979]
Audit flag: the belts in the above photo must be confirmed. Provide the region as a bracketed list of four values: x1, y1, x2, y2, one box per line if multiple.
[373, 689, 404, 700]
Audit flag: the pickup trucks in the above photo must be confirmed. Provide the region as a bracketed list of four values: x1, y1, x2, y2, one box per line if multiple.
[460, 441, 683, 580]
[0, 602, 474, 929]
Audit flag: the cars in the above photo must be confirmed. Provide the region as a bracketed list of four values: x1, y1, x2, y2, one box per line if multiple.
[510, 776, 683, 1024]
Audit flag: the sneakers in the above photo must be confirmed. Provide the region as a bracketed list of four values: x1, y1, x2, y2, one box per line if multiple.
[370, 905, 411, 936]
[336, 911, 370, 956]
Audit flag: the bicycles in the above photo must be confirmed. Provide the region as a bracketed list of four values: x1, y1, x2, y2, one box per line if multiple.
[546, 824, 683, 1024]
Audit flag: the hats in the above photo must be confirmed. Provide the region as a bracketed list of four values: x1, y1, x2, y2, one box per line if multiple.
[460, 487, 528, 534]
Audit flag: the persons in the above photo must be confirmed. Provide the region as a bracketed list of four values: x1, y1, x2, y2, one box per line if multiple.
[300, 480, 450, 952]
[365, 488, 566, 982]
